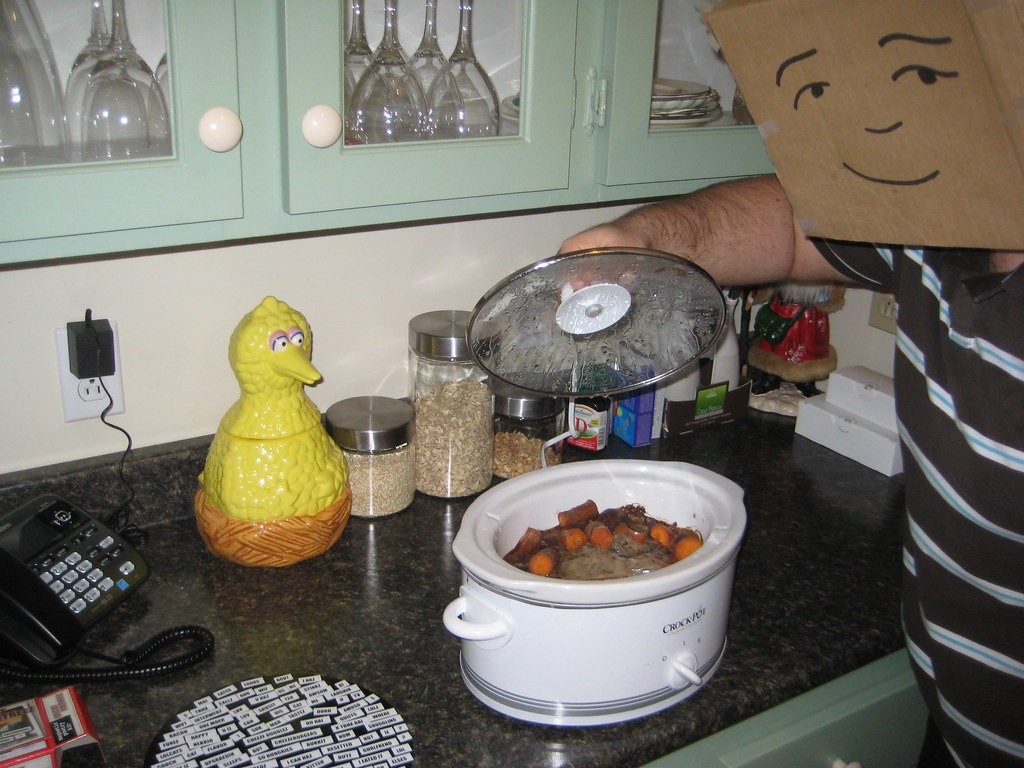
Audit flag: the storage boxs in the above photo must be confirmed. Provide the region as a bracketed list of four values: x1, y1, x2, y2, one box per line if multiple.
[0, 683, 107, 768]
[660, 357, 753, 441]
[611, 383, 656, 448]
[793, 392, 905, 477]
[826, 364, 901, 435]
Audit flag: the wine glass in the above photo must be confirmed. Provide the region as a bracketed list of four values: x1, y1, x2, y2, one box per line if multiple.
[1, 0, 503, 171]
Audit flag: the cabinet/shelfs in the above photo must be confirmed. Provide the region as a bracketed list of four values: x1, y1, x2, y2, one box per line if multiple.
[595, 0, 777, 204]
[0, 0, 584, 264]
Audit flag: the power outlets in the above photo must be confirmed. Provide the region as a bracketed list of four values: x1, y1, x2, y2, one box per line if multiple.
[867, 290, 898, 336]
[53, 320, 125, 424]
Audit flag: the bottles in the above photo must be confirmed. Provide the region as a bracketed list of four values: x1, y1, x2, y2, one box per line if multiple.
[488, 373, 564, 480]
[407, 310, 495, 498]
[325, 395, 417, 519]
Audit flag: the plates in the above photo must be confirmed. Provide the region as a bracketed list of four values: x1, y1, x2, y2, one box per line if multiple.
[503, 78, 724, 134]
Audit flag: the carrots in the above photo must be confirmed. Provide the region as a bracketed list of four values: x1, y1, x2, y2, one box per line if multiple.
[501, 497, 706, 576]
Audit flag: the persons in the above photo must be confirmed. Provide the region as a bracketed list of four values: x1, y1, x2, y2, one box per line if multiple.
[556, 171, 1024, 768]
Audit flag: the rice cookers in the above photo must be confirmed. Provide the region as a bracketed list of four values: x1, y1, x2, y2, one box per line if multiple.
[443, 459, 748, 728]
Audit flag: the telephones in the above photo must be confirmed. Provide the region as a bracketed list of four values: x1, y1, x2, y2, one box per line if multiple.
[0, 495, 149, 673]
